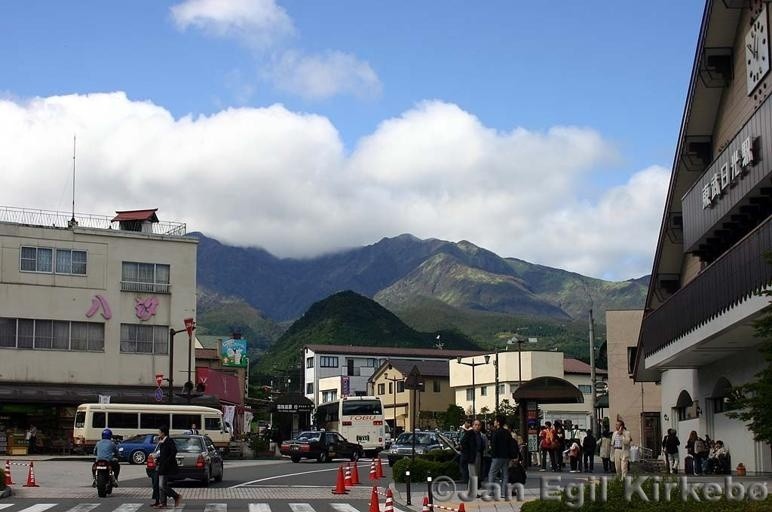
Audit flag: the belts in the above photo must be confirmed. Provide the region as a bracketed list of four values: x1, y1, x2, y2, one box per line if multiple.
[476, 450, 481, 452]
[614, 446, 622, 450]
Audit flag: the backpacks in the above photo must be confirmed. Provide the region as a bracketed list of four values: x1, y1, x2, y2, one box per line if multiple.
[504, 432, 518, 459]
[544, 428, 555, 445]
[693, 438, 705, 454]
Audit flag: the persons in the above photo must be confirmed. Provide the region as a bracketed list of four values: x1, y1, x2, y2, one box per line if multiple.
[455, 415, 528, 498]
[188, 424, 199, 435]
[92, 429, 120, 488]
[30, 424, 37, 455]
[153, 427, 182, 508]
[150, 436, 163, 507]
[662, 429, 728, 478]
[538, 419, 639, 481]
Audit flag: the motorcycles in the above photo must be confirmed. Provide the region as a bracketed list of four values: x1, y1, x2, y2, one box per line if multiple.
[93, 448, 124, 498]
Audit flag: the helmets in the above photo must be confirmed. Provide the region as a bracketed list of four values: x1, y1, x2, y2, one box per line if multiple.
[102, 429, 112, 440]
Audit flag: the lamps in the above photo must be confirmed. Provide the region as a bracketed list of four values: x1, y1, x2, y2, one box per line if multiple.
[697, 406, 702, 415]
[664, 414, 669, 421]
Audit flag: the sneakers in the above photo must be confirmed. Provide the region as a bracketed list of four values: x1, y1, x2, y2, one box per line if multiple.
[552, 467, 595, 473]
[92, 478, 97, 488]
[111, 475, 118, 488]
[174, 493, 182, 507]
[672, 467, 677, 475]
[152, 502, 168, 509]
[538, 468, 546, 472]
[150, 503, 160, 508]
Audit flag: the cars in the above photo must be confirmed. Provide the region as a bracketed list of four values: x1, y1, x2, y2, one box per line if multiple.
[280, 428, 365, 464]
[384, 419, 462, 462]
[114, 432, 161, 465]
[257, 420, 265, 426]
[144, 434, 225, 486]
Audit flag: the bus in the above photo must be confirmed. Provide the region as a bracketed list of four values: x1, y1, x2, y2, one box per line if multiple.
[71, 403, 236, 455]
[313, 395, 387, 459]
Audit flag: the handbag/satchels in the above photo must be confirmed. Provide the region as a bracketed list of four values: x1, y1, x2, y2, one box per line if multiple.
[145, 466, 156, 477]
[663, 446, 667, 453]
[507, 461, 527, 485]
[146, 454, 157, 469]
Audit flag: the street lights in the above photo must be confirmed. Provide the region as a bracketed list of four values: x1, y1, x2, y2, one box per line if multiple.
[455, 353, 491, 421]
[436, 334, 441, 350]
[505, 335, 526, 387]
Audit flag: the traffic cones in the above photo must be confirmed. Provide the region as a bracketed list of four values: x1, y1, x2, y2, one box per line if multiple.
[420, 496, 433, 512]
[20, 460, 42, 488]
[456, 503, 468, 512]
[325, 456, 398, 512]
[3, 458, 16, 485]
